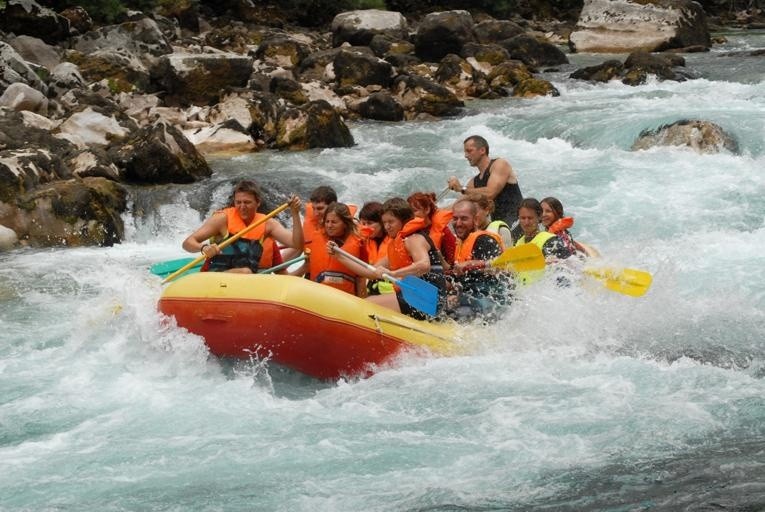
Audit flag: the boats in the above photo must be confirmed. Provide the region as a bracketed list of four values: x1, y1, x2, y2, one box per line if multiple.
[154, 236, 604, 385]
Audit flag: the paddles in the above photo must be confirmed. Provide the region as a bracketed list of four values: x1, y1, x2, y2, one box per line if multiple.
[583, 268, 652, 297]
[463, 243, 545, 272]
[151, 258, 205, 281]
[332, 246, 439, 316]
